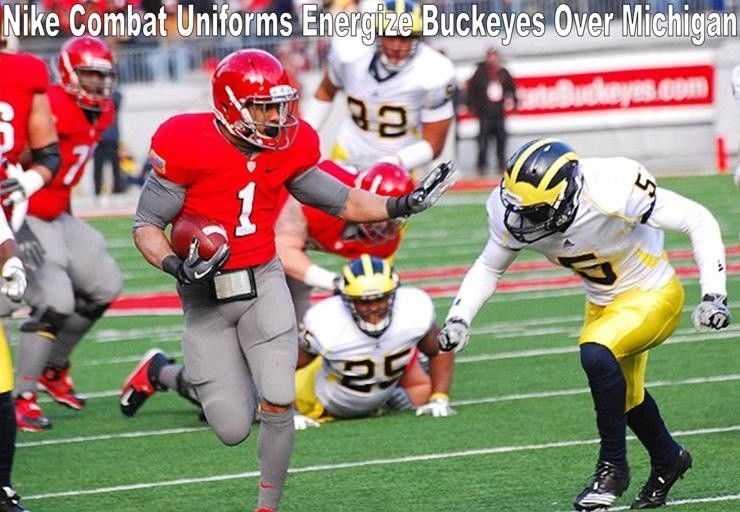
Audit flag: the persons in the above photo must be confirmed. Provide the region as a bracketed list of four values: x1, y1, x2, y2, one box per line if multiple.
[0, 50, 63, 320]
[439, 135, 732, 510]
[273, 158, 418, 367]
[306, 0, 460, 173]
[466, 47, 517, 174]
[132, 45, 460, 512]
[94, 88, 123, 194]
[11, 35, 126, 432]
[291, 249, 455, 433]
[0, 202, 27, 512]
[118, 349, 433, 415]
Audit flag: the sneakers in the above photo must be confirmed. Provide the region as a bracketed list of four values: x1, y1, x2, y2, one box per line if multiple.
[13, 388, 56, 431]
[572, 457, 632, 512]
[38, 361, 86, 412]
[631, 446, 694, 510]
[116, 346, 176, 419]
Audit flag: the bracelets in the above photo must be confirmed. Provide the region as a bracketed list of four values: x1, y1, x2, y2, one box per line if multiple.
[303, 263, 335, 290]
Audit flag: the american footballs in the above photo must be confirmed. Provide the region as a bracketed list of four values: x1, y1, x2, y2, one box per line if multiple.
[171, 213, 229, 262]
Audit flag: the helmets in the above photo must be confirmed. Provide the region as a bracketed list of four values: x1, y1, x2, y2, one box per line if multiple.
[332, 254, 401, 338]
[209, 46, 303, 151]
[52, 32, 118, 115]
[373, 0, 427, 69]
[356, 159, 417, 249]
[497, 138, 586, 247]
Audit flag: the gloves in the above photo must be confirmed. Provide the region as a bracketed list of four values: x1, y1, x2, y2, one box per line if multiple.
[385, 159, 460, 221]
[9, 218, 46, 273]
[0, 165, 44, 208]
[161, 237, 232, 287]
[434, 317, 471, 355]
[294, 414, 320, 431]
[0, 254, 30, 298]
[691, 292, 732, 335]
[414, 392, 459, 419]
